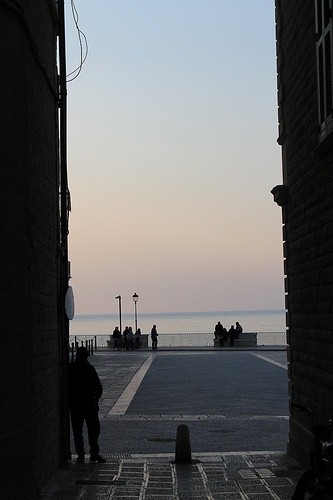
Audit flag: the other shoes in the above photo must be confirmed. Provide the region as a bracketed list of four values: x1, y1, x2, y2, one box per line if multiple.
[90, 455, 106, 463]
[74, 457, 84, 463]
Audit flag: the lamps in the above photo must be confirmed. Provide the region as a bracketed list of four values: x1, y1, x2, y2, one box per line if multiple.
[272, 184, 289, 205]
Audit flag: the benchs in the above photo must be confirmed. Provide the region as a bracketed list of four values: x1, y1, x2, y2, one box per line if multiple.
[107, 335, 147, 347]
[214, 333, 257, 347]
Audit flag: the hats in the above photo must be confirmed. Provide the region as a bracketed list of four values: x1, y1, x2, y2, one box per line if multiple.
[77, 347, 90, 356]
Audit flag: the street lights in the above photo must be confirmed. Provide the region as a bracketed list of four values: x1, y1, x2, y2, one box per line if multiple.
[131, 292, 140, 331]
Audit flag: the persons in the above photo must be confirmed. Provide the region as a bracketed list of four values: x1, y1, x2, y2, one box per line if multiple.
[112, 327, 141, 351]
[229, 325, 235, 347]
[236, 322, 242, 339]
[67, 347, 106, 463]
[214, 322, 228, 342]
[151, 325, 158, 349]
